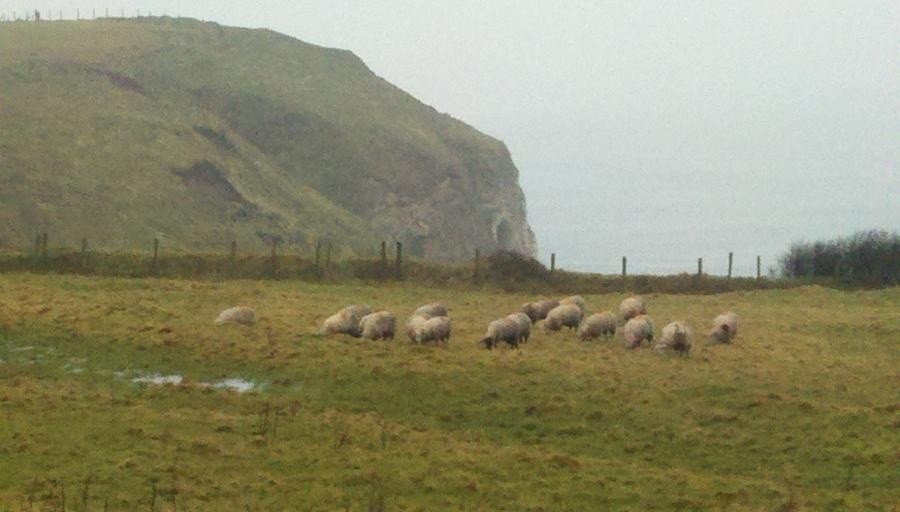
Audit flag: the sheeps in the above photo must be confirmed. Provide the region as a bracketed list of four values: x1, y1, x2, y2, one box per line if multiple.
[214, 305, 260, 327]
[325, 304, 454, 347]
[478, 295, 742, 360]
